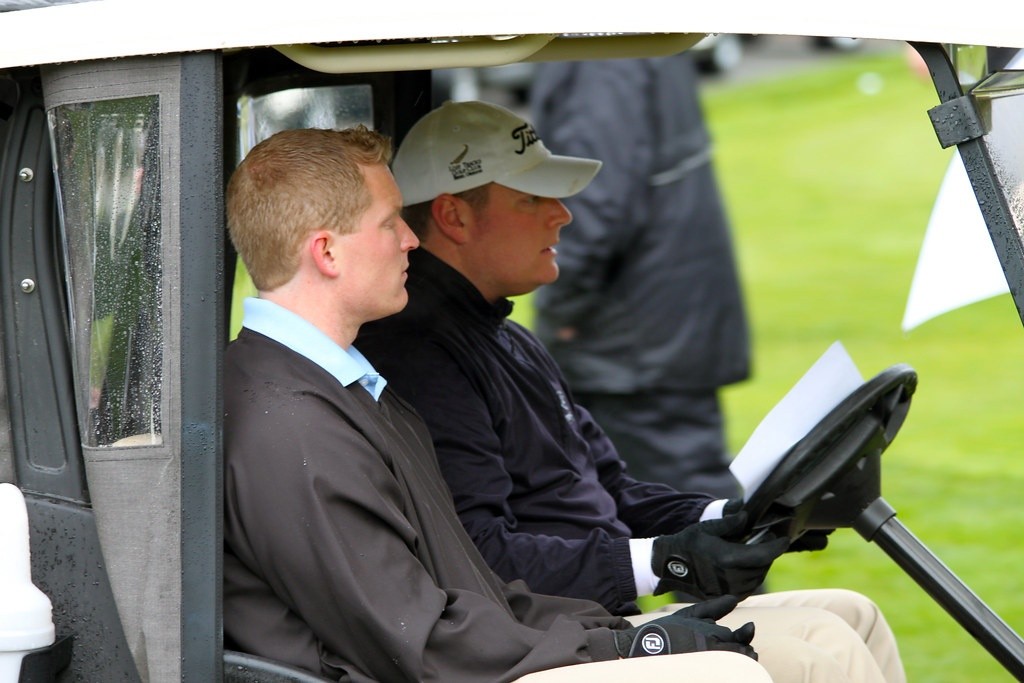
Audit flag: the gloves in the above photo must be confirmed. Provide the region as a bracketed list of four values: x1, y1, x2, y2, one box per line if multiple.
[611, 593, 758, 663]
[651, 495, 791, 598]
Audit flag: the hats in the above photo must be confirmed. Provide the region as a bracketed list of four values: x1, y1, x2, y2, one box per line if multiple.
[395, 100, 603, 208]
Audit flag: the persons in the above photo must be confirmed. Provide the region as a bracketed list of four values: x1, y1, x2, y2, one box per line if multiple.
[525, 54, 756, 505]
[352, 100, 907, 683]
[224, 125, 777, 683]
